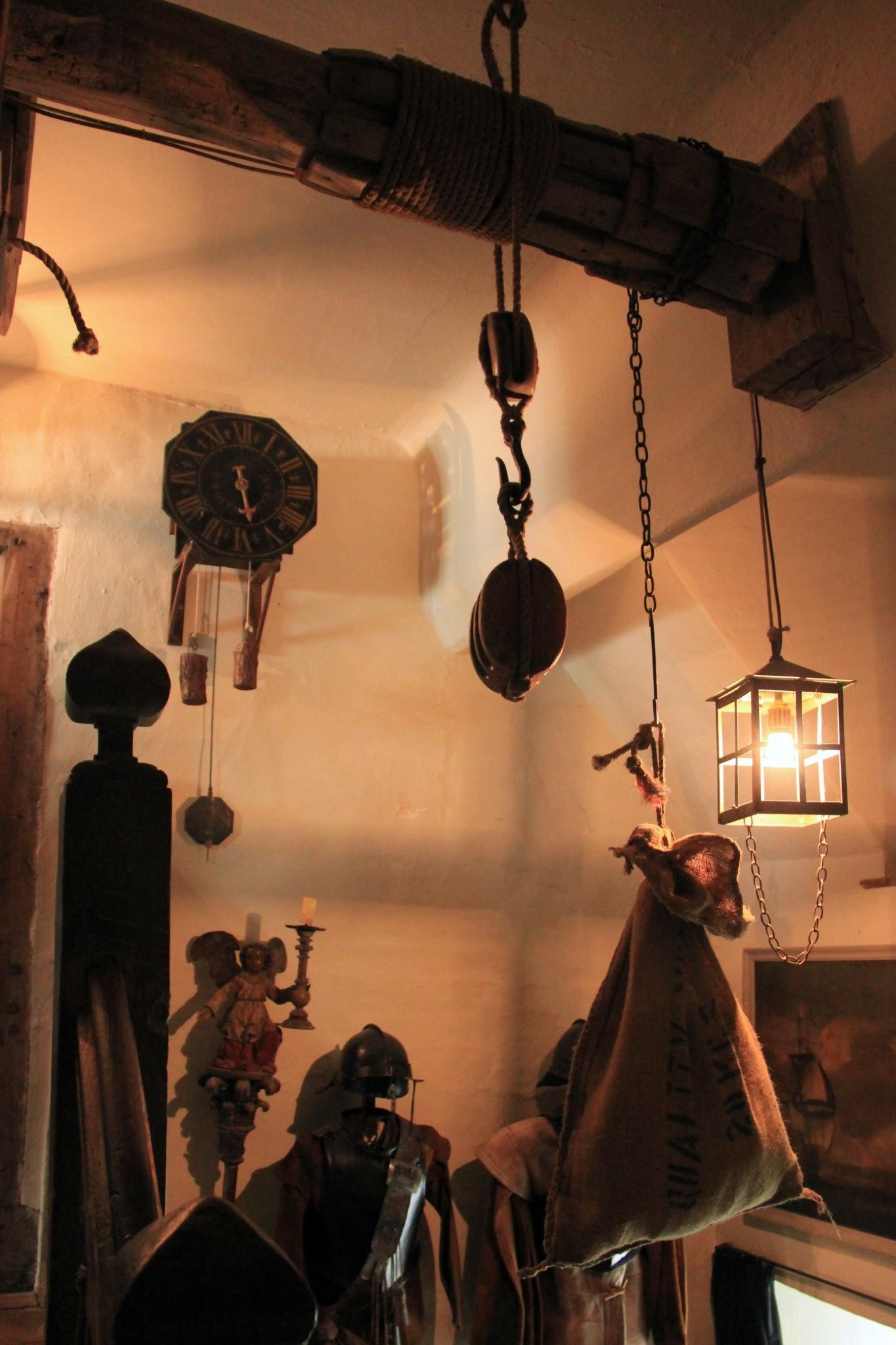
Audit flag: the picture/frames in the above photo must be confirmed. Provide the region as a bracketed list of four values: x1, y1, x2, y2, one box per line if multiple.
[741, 943, 896, 1269]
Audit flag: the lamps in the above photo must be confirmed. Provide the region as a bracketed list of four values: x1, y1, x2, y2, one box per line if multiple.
[703, 385, 860, 965]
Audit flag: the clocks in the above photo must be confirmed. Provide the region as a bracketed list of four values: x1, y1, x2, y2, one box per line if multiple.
[160, 409, 319, 863]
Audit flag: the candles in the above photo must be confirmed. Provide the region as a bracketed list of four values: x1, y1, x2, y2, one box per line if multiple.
[300, 895, 317, 925]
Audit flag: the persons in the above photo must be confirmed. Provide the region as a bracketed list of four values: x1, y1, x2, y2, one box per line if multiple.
[196, 935, 311, 1077]
